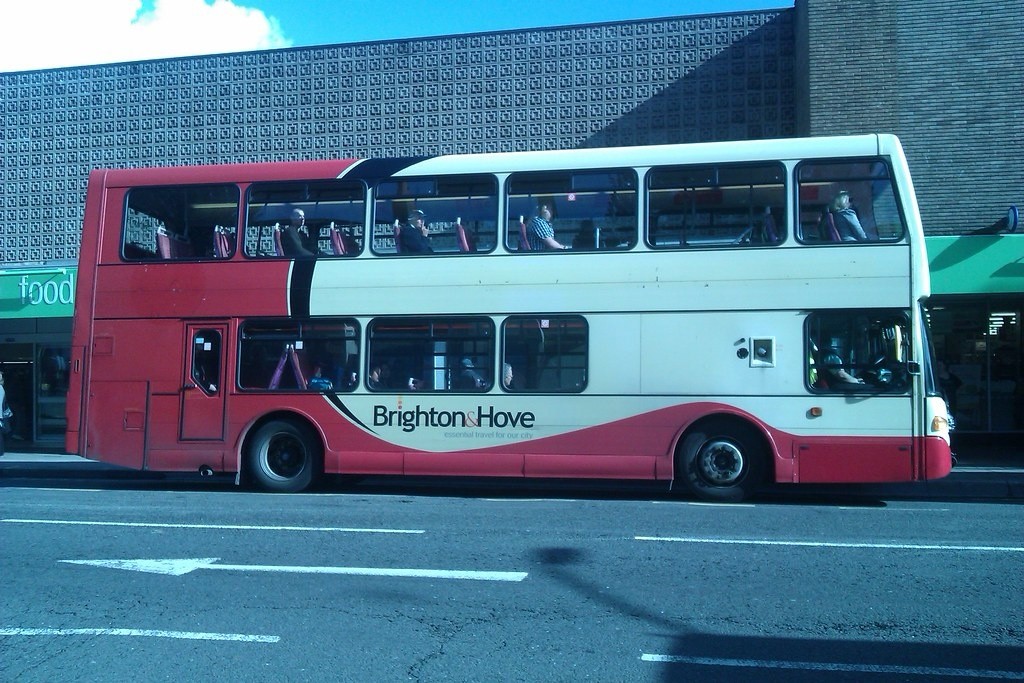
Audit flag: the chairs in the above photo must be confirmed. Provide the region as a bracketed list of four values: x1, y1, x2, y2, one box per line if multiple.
[274, 223, 285, 256]
[519, 215, 532, 251]
[817, 213, 828, 243]
[393, 219, 402, 255]
[214, 225, 229, 257]
[157, 225, 191, 259]
[330, 221, 348, 256]
[456, 217, 474, 252]
[823, 204, 842, 242]
[763, 206, 779, 243]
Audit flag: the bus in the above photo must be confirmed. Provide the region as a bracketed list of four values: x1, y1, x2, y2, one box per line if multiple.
[64, 132, 958, 503]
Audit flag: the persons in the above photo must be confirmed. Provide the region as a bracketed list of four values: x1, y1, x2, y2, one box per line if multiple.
[399, 209, 433, 255]
[813, 334, 865, 387]
[520, 199, 572, 251]
[281, 209, 328, 257]
[0, 371, 25, 457]
[822, 184, 879, 241]
[308, 358, 515, 392]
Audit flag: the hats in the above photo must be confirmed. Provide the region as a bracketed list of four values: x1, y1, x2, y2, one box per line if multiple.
[407, 209, 427, 220]
[460, 359, 474, 368]
